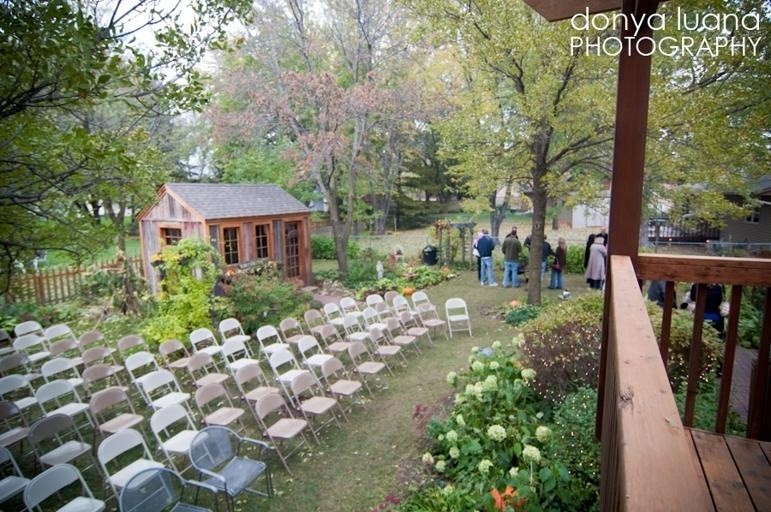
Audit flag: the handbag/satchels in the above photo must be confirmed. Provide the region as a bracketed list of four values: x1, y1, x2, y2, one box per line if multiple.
[551, 258, 560, 270]
[718, 284, 730, 317]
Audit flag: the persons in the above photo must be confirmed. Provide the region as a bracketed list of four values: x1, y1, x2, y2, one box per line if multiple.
[523, 234, 531, 250]
[507, 226, 518, 239]
[549, 237, 567, 290]
[472, 232, 483, 282]
[648, 280, 677, 309]
[683, 283, 731, 334]
[584, 226, 608, 290]
[540, 234, 552, 284]
[502, 230, 522, 289]
[477, 227, 499, 288]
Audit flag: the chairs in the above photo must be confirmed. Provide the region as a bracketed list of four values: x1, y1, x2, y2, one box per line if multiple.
[445, 297, 472, 338]
